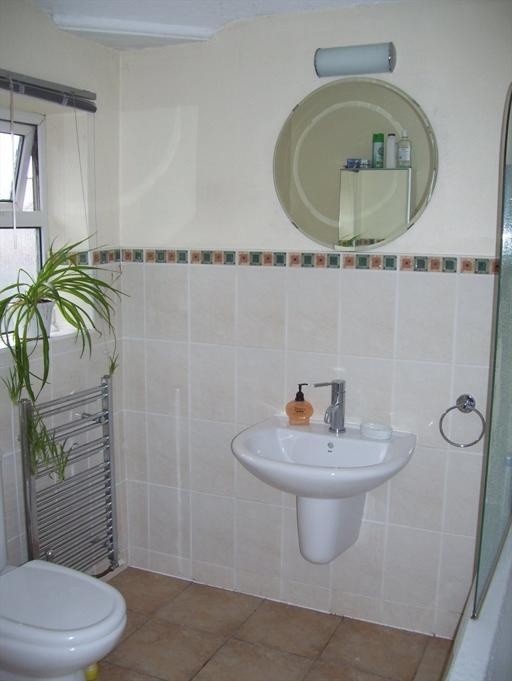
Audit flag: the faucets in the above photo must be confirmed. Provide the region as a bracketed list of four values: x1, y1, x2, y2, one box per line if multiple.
[312, 380, 347, 432]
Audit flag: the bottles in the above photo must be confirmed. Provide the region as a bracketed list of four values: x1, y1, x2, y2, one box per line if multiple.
[372, 129, 412, 169]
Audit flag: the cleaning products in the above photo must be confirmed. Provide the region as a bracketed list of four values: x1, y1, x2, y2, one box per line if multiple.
[373, 130, 412, 168]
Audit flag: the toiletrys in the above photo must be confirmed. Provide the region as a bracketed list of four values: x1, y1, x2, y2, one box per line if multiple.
[286, 384, 314, 427]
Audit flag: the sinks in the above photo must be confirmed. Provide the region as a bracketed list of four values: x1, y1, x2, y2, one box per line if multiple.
[231, 416, 418, 500]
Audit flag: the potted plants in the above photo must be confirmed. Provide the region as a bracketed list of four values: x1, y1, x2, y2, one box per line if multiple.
[0, 230, 131, 481]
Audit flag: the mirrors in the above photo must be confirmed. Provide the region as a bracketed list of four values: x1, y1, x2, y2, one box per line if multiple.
[272, 77, 438, 252]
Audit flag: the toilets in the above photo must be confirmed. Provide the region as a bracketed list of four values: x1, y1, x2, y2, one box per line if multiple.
[0, 486, 128, 681]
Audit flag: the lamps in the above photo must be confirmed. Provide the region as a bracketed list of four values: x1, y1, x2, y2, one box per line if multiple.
[314, 42, 397, 79]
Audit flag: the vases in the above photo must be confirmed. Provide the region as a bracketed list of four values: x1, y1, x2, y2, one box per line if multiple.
[14, 300, 54, 338]
